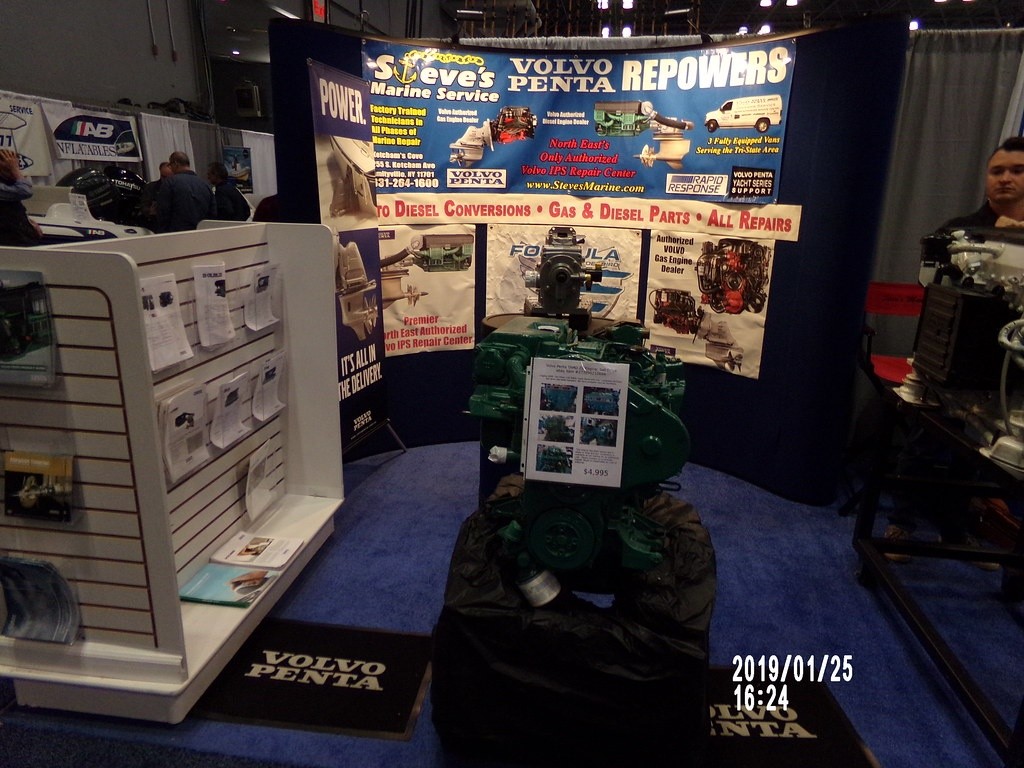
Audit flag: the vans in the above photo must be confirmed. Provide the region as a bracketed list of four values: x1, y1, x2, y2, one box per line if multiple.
[705, 95, 783, 133]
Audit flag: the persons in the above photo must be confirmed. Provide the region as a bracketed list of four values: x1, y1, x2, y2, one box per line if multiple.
[148, 150, 214, 234]
[882, 136, 1024, 570]
[206, 162, 250, 222]
[0, 149, 42, 243]
[140, 161, 174, 228]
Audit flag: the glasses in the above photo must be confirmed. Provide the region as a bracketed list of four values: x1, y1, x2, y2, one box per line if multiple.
[167, 162, 172, 169]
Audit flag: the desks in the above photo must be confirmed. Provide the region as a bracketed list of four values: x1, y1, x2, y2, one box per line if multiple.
[855, 291, 1024, 768]
